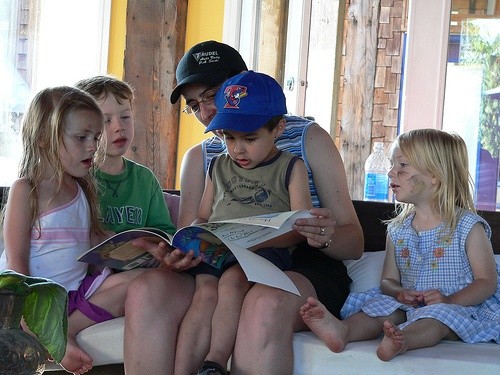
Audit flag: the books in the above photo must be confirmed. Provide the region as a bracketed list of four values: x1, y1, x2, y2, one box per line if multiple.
[77, 208, 313, 299]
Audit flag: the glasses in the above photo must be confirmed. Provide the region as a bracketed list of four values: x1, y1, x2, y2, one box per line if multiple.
[182, 90, 216, 116]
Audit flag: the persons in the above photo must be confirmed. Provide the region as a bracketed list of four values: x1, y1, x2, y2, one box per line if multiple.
[300, 129, 500, 361]
[125, 40, 364, 375]
[73, 76, 177, 272]
[174, 71, 313, 375]
[0, 87, 143, 375]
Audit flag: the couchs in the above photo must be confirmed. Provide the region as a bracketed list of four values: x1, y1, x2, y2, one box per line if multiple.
[0, 187, 500, 375]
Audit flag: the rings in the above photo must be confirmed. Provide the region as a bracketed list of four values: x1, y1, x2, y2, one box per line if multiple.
[320, 227, 325, 235]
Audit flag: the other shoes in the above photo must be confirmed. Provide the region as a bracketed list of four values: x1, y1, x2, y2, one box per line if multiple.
[198, 360, 231, 375]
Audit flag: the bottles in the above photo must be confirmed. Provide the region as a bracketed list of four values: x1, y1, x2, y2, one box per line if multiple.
[363, 142, 391, 202]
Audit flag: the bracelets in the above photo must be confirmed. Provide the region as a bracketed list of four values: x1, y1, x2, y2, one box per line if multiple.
[320, 239, 333, 249]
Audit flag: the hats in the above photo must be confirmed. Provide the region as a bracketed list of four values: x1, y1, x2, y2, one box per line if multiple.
[204, 70, 287, 133]
[170, 40, 248, 104]
